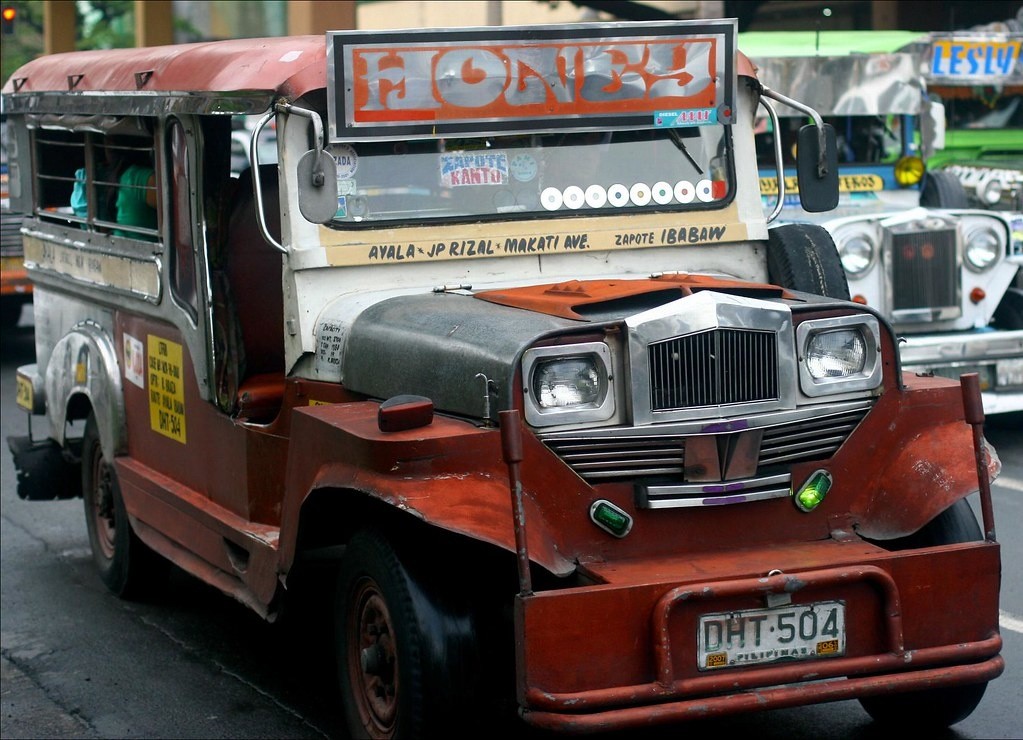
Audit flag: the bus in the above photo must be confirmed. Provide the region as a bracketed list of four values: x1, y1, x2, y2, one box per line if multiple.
[0, 16, 1006, 740]
[739, 32, 1022, 302]
[735, 48, 1021, 429]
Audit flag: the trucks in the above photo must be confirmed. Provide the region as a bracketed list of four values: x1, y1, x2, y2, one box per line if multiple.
[0, 116, 35, 334]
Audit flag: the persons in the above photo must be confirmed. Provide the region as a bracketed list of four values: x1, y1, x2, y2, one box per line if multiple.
[70, 168, 88, 230]
[113, 142, 157, 243]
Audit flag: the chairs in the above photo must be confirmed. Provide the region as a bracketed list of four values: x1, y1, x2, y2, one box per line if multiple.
[227, 185, 284, 421]
